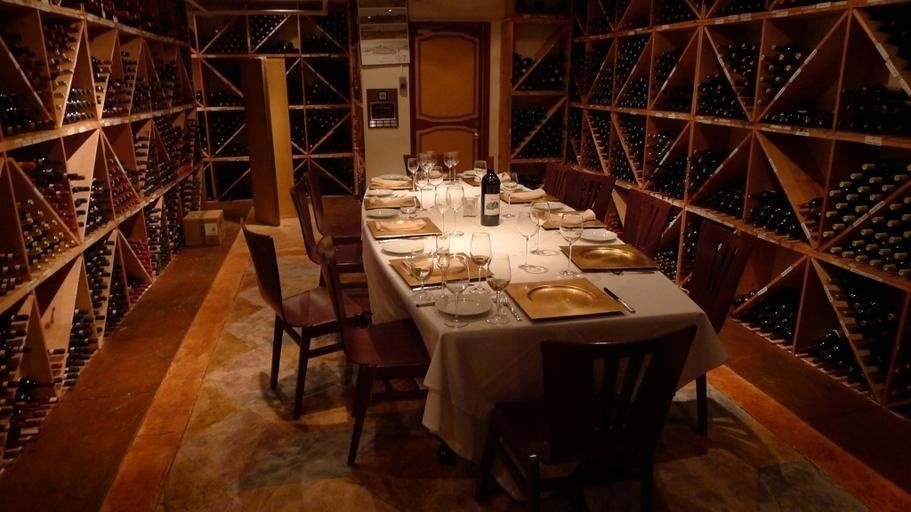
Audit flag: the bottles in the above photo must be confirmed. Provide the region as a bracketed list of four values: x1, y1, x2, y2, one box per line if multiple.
[566, 4, 910, 417]
[481, 157, 501, 225]
[0, 13, 197, 483]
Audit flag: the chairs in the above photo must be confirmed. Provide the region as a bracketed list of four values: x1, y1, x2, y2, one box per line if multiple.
[474, 326, 698, 509]
[681, 217, 754, 456]
[543, 161, 574, 200]
[240, 223, 367, 421]
[620, 188, 673, 256]
[302, 168, 360, 244]
[291, 181, 361, 287]
[564, 169, 617, 221]
[314, 229, 452, 469]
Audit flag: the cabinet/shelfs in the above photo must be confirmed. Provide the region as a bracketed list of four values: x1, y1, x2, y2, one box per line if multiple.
[0, 0, 206, 512]
[188, 0, 365, 206]
[498, 0, 911, 496]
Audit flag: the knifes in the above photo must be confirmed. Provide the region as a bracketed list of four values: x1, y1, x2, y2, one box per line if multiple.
[604, 287, 638, 314]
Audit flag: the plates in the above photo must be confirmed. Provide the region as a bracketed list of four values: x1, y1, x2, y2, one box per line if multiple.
[579, 229, 617, 241]
[365, 207, 399, 218]
[364, 188, 391, 197]
[381, 240, 424, 254]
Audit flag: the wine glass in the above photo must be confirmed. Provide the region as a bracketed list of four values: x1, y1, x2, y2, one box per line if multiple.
[408, 149, 467, 237]
[408, 231, 511, 328]
[501, 172, 519, 219]
[517, 199, 583, 280]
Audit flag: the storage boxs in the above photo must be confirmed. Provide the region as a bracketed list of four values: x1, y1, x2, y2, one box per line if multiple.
[183, 209, 226, 246]
